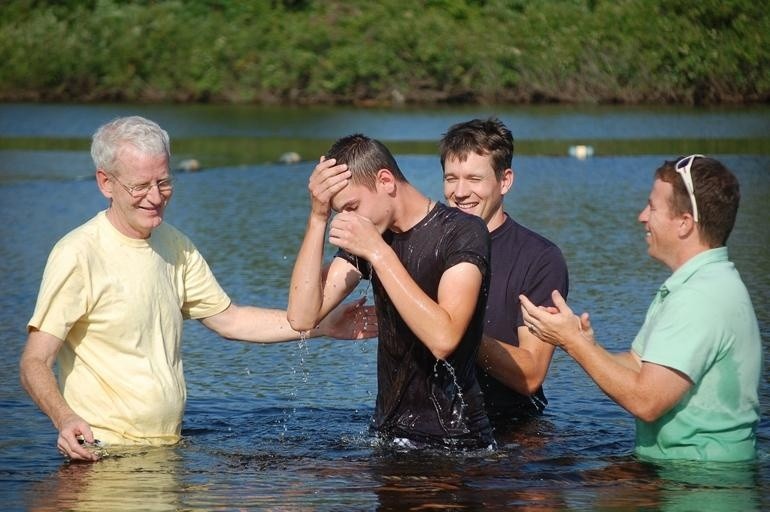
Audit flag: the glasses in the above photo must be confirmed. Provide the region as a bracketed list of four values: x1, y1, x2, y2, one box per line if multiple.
[675, 154, 709, 223]
[99, 164, 176, 199]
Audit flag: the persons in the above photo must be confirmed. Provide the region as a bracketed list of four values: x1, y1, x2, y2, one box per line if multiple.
[439, 117, 568, 427]
[286, 134, 497, 454]
[18, 116, 380, 462]
[518, 157, 763, 464]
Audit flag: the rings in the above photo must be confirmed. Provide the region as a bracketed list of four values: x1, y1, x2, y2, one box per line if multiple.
[529, 325, 534, 333]
